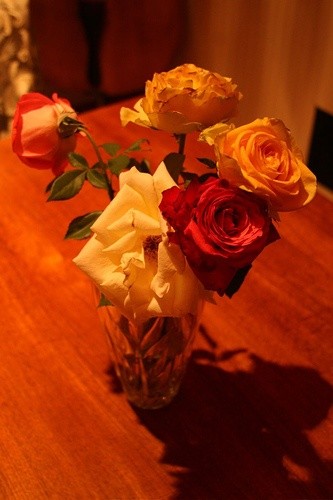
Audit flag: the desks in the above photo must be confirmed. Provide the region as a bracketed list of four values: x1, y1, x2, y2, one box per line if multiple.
[0, 97, 333, 499]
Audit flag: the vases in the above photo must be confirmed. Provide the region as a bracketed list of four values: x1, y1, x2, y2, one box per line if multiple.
[92, 296, 200, 411]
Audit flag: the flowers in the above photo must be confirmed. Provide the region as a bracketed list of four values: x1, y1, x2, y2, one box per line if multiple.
[11, 62, 318, 398]
